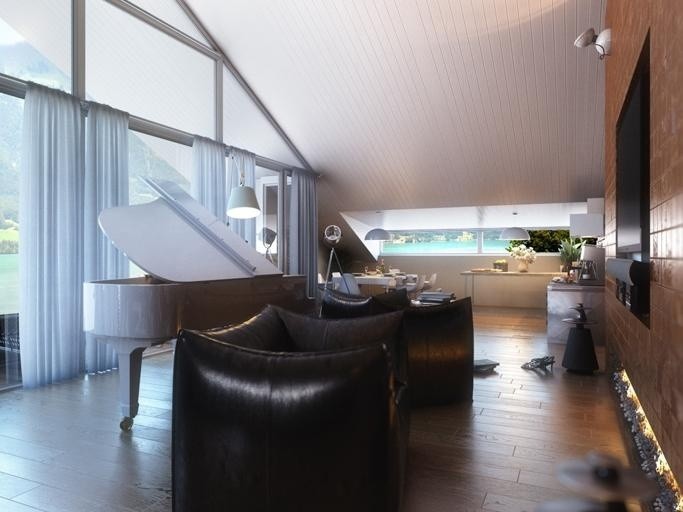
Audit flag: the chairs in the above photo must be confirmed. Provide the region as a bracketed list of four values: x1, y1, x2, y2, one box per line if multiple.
[170, 301, 412, 512]
[317, 270, 436, 296]
[319, 293, 475, 406]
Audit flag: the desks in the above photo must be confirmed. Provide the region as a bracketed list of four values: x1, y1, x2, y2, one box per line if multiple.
[460, 271, 568, 306]
[546, 280, 606, 345]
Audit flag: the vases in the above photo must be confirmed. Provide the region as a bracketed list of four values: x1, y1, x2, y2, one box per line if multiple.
[519, 261, 528, 272]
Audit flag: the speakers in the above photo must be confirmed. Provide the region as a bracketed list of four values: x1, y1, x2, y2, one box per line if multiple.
[606, 258, 638, 287]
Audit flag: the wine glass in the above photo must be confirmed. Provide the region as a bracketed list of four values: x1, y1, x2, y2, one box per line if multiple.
[364, 266, 368, 276]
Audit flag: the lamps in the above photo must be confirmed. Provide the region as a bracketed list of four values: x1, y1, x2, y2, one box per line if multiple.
[364, 212, 391, 241]
[580, 245, 597, 279]
[498, 228, 529, 240]
[227, 183, 260, 219]
[574, 28, 612, 59]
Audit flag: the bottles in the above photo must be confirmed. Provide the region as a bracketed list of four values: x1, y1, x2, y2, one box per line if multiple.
[381, 259, 385, 274]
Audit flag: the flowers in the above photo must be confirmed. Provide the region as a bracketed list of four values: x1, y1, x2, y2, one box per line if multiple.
[511, 244, 536, 264]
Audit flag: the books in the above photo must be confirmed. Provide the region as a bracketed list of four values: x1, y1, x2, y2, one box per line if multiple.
[416, 291, 456, 307]
[470, 268, 503, 272]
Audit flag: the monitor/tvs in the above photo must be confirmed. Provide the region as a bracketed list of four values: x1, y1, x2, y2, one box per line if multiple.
[615, 25, 650, 253]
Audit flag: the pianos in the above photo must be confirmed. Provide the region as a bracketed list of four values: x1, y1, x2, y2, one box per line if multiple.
[81, 178, 307, 430]
